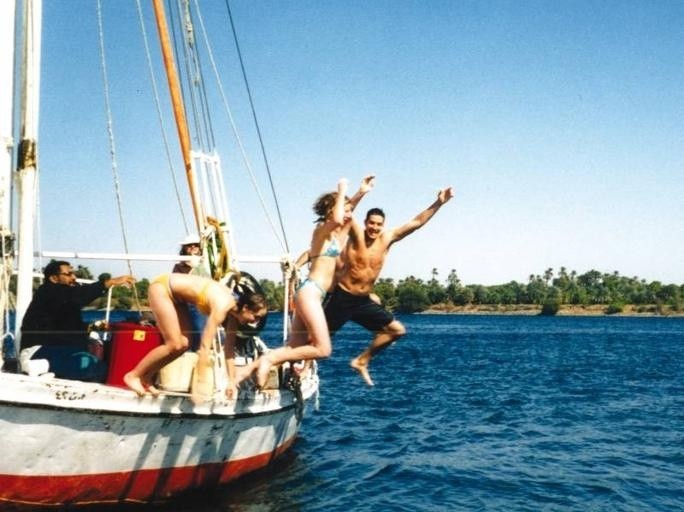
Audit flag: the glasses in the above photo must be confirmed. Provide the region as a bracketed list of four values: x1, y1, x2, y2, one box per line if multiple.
[54, 272, 73, 276]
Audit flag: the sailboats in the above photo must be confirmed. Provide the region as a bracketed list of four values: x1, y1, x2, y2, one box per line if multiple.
[1, 0, 320, 508]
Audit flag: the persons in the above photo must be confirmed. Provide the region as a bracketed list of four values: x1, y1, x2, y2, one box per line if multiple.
[168, 233, 201, 273]
[18, 260, 138, 380]
[292, 174, 456, 387]
[120, 270, 270, 399]
[251, 179, 378, 392]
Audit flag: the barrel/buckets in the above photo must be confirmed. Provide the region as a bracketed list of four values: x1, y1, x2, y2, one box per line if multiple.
[161, 352, 198, 395]
[109, 323, 160, 392]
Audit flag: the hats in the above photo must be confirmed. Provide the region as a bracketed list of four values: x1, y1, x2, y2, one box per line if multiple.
[180, 234, 200, 245]
